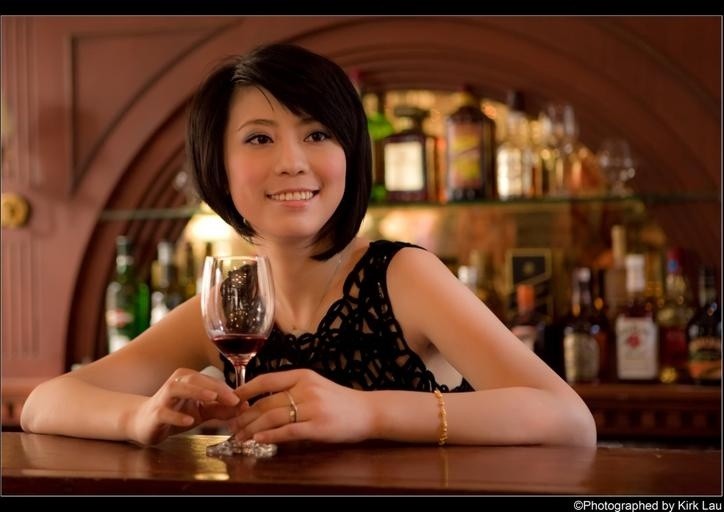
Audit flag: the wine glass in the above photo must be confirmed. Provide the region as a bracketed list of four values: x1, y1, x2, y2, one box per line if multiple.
[200, 256, 279, 458]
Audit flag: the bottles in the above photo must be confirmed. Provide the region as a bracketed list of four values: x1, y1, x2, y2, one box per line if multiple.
[456, 226, 722, 387]
[364, 83, 635, 205]
[107, 233, 214, 355]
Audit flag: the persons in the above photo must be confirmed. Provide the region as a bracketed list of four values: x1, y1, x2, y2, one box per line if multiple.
[17, 42, 599, 450]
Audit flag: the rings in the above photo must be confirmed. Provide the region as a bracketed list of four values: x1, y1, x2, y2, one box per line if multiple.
[285, 404, 297, 423]
[281, 389, 295, 407]
[174, 375, 183, 383]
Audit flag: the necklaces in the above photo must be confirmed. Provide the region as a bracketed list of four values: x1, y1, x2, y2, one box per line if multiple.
[264, 244, 344, 333]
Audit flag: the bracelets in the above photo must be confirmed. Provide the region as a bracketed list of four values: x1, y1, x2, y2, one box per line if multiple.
[431, 384, 449, 447]
